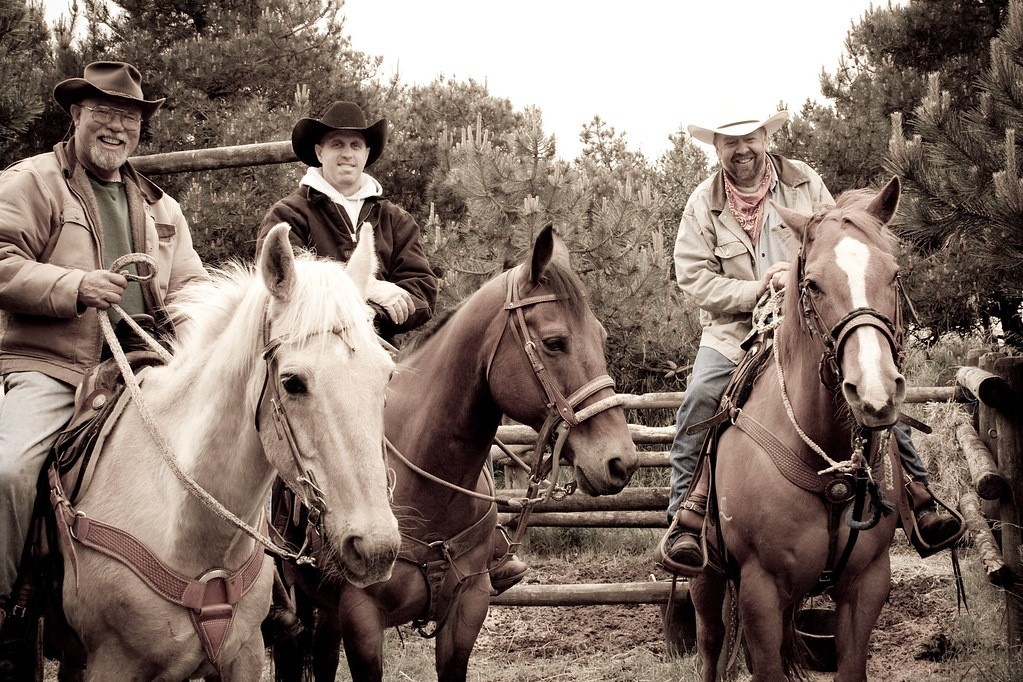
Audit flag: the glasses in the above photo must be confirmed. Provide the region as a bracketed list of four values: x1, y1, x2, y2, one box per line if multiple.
[76, 104, 143, 131]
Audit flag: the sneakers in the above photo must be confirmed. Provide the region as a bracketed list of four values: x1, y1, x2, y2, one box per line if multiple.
[489, 560, 531, 589]
[260, 605, 305, 647]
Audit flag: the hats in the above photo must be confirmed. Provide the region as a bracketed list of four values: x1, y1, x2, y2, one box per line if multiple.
[51, 61, 167, 128]
[686, 100, 789, 145]
[290, 100, 387, 169]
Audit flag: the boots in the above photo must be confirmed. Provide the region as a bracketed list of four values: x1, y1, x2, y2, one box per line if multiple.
[655, 516, 703, 579]
[899, 477, 961, 559]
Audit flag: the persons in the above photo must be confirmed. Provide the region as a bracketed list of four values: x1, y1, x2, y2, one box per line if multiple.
[248, 100, 441, 596]
[1, 57, 303, 656]
[650, 95, 969, 572]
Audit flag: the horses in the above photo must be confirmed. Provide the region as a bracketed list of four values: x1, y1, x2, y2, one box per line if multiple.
[662, 172, 907, 682]
[262, 220, 641, 682]
[0, 220, 402, 682]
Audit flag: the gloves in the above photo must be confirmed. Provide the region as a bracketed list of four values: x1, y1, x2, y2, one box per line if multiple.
[368, 281, 416, 325]
